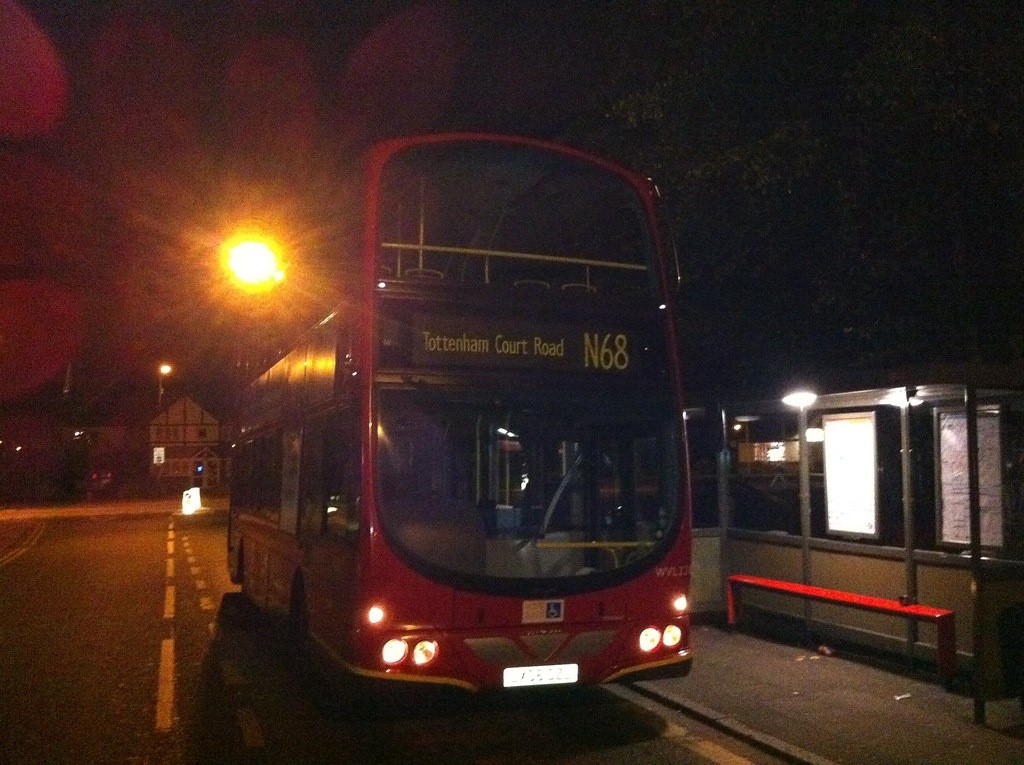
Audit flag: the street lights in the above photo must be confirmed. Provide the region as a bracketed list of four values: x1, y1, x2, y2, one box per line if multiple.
[782, 388, 824, 637]
[158, 359, 173, 404]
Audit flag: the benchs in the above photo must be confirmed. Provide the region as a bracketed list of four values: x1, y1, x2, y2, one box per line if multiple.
[723, 573, 957, 683]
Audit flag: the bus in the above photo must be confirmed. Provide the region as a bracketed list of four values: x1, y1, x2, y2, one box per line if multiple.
[223, 127, 697, 711]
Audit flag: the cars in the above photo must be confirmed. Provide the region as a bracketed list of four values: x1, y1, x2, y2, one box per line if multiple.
[83, 469, 114, 494]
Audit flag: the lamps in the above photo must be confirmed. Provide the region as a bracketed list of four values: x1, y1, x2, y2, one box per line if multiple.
[781, 390, 817, 409]
